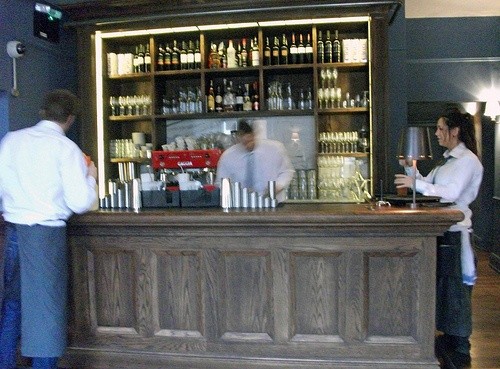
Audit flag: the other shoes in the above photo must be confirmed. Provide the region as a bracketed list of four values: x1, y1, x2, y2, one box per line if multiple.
[435, 334, 472, 369]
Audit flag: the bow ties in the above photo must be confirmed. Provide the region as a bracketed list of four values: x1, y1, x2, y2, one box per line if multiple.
[435, 156, 453, 165]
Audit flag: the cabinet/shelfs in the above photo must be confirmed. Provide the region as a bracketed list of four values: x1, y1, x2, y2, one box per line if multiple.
[75, 0, 399, 201]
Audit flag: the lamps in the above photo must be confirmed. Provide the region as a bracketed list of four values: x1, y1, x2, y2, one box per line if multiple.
[396, 126, 434, 208]
[484, 96, 500, 125]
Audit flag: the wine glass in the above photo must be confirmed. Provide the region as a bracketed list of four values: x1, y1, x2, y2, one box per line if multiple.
[317, 68, 341, 109]
[359, 138, 369, 154]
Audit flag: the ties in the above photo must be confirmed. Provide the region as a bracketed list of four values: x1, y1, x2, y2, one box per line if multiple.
[246, 154, 254, 188]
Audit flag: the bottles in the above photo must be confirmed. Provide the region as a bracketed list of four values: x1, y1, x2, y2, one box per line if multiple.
[109, 96, 152, 116]
[207, 37, 259, 68]
[317, 30, 341, 63]
[357, 128, 367, 152]
[263, 31, 312, 66]
[109, 139, 140, 158]
[205, 78, 260, 112]
[318, 131, 359, 153]
[156, 39, 201, 71]
[132, 44, 151, 74]
[266, 81, 312, 111]
[161, 86, 203, 115]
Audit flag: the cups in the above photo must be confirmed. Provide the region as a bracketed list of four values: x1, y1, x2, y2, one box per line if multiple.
[132, 132, 145, 146]
[221, 177, 277, 208]
[396, 184, 408, 196]
[285, 170, 317, 199]
[342, 38, 368, 63]
[106, 52, 132, 75]
[317, 156, 359, 200]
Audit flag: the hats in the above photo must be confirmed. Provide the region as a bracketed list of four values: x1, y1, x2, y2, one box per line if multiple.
[231, 119, 254, 133]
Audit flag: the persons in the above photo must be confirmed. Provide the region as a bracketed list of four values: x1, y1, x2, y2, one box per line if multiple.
[0, 89, 99, 369]
[394, 108, 486, 369]
[215, 118, 295, 208]
